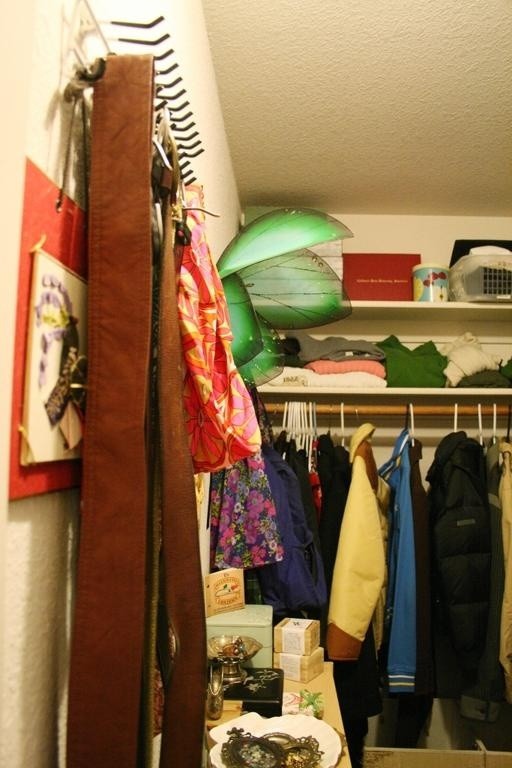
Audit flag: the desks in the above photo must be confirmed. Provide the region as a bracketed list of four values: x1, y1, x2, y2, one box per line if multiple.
[193, 623, 352, 768]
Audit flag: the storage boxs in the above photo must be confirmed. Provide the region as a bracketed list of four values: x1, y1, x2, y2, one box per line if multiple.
[362, 694, 486, 766]
[272, 647, 326, 685]
[453, 693, 512, 768]
[271, 613, 320, 655]
[204, 603, 273, 668]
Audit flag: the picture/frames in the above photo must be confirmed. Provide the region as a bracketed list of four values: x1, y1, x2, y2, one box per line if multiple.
[19, 245, 87, 467]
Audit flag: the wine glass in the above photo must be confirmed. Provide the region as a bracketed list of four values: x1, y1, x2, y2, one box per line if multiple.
[206, 633, 263, 682]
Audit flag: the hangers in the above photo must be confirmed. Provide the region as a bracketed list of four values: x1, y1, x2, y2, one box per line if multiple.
[268, 402, 511, 472]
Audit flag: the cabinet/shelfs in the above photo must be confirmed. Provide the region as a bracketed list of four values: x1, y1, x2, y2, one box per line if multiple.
[64, 12, 218, 768]
[252, 298, 512, 397]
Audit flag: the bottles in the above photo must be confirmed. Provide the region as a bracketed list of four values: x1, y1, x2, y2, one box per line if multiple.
[410, 262, 450, 303]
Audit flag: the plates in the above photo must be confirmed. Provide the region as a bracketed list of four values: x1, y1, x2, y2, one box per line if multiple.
[209, 713, 344, 768]
